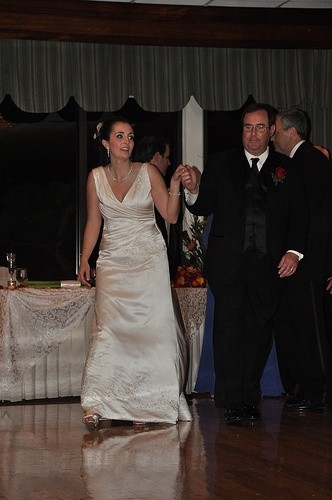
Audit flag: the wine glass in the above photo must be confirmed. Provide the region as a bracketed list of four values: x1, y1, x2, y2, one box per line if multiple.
[16, 267, 29, 288]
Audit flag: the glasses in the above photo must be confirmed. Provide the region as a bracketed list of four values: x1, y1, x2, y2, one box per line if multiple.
[243, 125, 269, 132]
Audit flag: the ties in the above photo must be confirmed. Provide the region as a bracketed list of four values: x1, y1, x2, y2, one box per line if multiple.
[250, 158, 259, 177]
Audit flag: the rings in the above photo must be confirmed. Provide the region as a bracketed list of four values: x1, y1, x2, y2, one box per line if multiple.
[78, 278, 80, 279]
[291, 269, 293, 271]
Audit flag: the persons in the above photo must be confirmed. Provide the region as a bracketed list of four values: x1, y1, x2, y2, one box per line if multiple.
[80, 425, 180, 500]
[78, 116, 192, 429]
[179, 104, 332, 423]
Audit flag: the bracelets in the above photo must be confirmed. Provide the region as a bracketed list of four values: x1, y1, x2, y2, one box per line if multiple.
[168, 190, 181, 197]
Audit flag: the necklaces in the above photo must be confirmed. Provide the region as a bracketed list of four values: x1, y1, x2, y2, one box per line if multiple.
[107, 160, 133, 182]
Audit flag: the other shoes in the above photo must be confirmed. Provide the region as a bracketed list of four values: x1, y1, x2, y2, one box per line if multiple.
[81, 413, 102, 427]
[133, 421, 145, 425]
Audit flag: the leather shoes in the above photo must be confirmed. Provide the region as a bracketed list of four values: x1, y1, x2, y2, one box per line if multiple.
[224, 406, 261, 424]
[282, 398, 324, 413]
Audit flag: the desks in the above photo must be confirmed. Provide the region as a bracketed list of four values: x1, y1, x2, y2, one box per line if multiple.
[0, 282, 207, 402]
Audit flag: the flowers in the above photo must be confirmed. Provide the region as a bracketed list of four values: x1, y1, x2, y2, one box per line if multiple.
[173, 214, 207, 289]
[273, 167, 286, 186]
